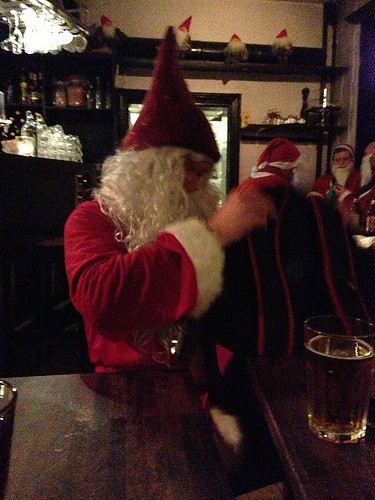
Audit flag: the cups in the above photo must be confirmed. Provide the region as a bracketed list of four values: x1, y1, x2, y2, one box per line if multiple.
[302, 314, 375, 444]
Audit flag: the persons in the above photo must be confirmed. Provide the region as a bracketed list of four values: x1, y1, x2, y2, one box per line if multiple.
[234, 136, 303, 213]
[272, 28, 293, 63]
[347, 142, 375, 239]
[224, 33, 249, 63]
[312, 143, 363, 229]
[62, 106, 295, 487]
[174, 16, 193, 52]
[100, 15, 126, 40]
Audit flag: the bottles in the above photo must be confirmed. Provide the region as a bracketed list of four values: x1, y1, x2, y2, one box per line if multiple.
[366, 199, 375, 234]
[0, 65, 107, 163]
[327, 180, 337, 202]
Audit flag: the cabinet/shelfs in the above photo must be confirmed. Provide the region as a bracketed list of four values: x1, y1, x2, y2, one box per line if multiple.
[0, 53, 119, 166]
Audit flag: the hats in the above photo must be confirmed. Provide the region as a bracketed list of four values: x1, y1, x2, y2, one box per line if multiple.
[115, 26, 221, 163]
[258, 137, 302, 171]
[330, 144, 354, 168]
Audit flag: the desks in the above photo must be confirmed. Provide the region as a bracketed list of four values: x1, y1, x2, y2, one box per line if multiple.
[1, 150, 93, 364]
[1, 369, 235, 498]
[243, 349, 374, 499]
